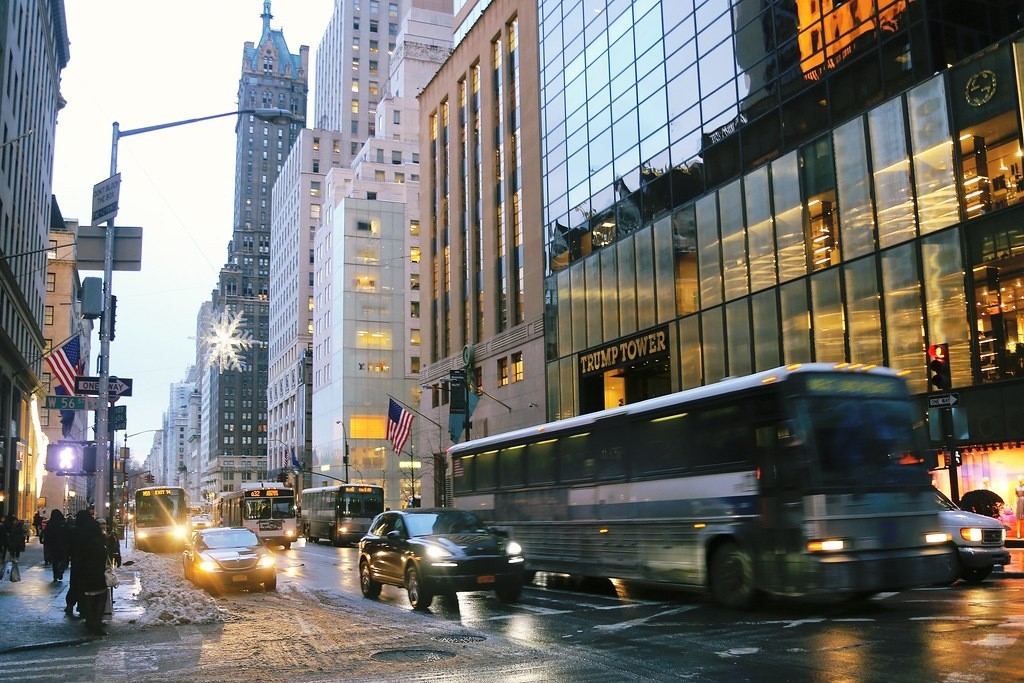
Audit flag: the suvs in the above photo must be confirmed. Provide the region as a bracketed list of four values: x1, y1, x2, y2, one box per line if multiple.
[358, 506, 528, 611]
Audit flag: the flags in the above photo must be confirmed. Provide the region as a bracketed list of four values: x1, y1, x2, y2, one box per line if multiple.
[448, 388, 480, 445]
[45, 334, 80, 397]
[445, 458, 463, 479]
[284, 449, 301, 469]
[55, 362, 85, 438]
[385, 398, 415, 456]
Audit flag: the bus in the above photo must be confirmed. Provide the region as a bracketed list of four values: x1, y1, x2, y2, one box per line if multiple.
[130, 486, 191, 552]
[443, 361, 966, 632]
[222, 485, 302, 550]
[300, 483, 391, 548]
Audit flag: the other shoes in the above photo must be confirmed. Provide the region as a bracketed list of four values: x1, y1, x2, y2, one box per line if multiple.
[58, 570, 63, 580]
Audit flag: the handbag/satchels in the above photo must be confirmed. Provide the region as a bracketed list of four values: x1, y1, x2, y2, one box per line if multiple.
[104, 568, 120, 587]
[10, 563, 20, 582]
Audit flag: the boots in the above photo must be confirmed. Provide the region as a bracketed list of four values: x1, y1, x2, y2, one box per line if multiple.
[82, 590, 109, 635]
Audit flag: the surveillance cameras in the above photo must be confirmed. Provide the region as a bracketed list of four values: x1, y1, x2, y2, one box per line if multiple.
[529, 403, 533, 408]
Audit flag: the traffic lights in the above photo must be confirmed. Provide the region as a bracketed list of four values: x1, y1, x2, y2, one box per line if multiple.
[286, 472, 295, 484]
[277, 473, 282, 482]
[928, 342, 952, 391]
[145, 475, 150, 483]
[45, 443, 80, 473]
[282, 473, 288, 482]
[150, 474, 155, 483]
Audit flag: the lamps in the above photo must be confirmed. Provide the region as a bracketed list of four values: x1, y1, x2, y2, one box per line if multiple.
[811, 233, 832, 265]
[965, 174, 986, 211]
[979, 338, 997, 372]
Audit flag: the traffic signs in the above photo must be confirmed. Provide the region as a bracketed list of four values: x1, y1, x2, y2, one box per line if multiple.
[74, 375, 133, 397]
[926, 393, 961, 410]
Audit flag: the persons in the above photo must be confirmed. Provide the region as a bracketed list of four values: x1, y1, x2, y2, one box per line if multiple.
[31, 508, 121, 612]
[0, 512, 30, 563]
[1015, 475, 1024, 539]
[261, 506, 270, 518]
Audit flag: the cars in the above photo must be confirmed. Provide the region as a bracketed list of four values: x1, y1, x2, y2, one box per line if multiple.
[183, 525, 278, 598]
[108, 499, 137, 526]
[185, 505, 218, 531]
[931, 485, 1012, 584]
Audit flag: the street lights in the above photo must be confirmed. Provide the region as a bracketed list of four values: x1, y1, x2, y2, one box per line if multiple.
[335, 420, 349, 484]
[91, 107, 304, 516]
[122, 429, 165, 524]
